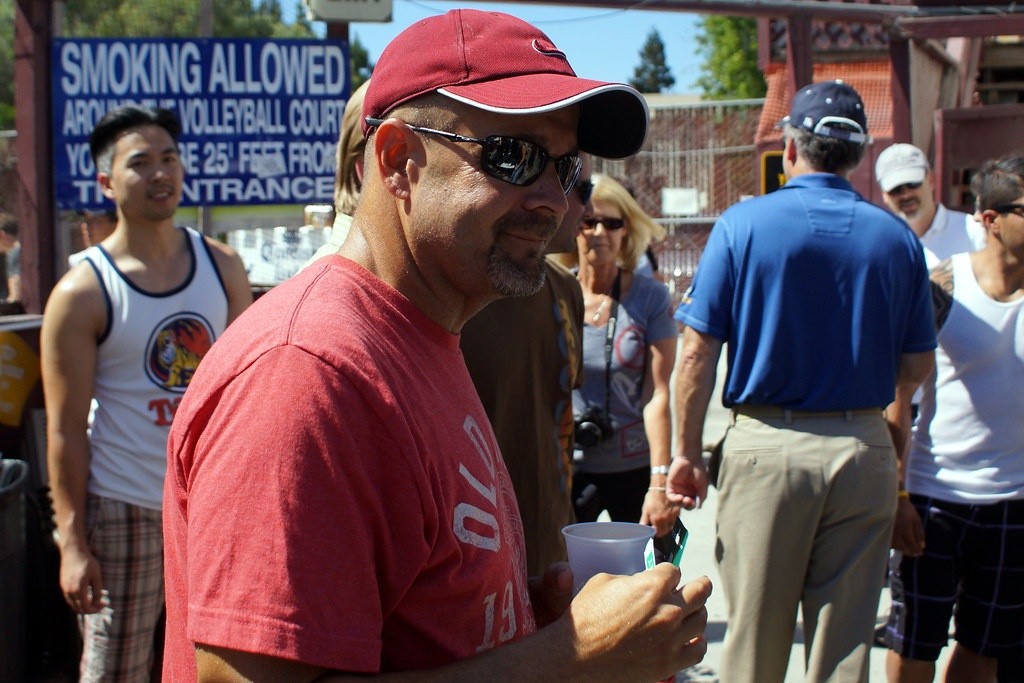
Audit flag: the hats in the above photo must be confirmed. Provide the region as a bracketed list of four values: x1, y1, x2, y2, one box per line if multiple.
[359, 6, 650, 163]
[873, 142, 928, 194]
[774, 79, 874, 148]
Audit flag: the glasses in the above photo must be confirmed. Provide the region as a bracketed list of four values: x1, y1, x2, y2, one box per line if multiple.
[889, 181, 925, 196]
[583, 216, 626, 231]
[363, 114, 584, 196]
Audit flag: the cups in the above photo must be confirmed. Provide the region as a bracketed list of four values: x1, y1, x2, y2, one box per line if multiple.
[562, 521, 657, 604]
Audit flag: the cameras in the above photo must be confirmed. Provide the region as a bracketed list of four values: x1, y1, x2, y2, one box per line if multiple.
[572, 408, 614, 447]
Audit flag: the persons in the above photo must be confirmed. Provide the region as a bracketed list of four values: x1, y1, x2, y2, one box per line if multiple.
[296, 79, 370, 274]
[461, 155, 598, 578]
[163, 8, 714, 683]
[0, 212, 23, 303]
[876, 144, 989, 269]
[567, 173, 681, 539]
[79, 211, 118, 247]
[667, 81, 939, 683]
[39, 105, 255, 682]
[884, 155, 1024, 683]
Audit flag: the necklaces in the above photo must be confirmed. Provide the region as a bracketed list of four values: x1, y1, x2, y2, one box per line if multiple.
[575, 277, 611, 322]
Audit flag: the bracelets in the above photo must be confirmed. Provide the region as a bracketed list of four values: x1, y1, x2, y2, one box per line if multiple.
[651, 465, 670, 474]
[648, 487, 665, 490]
[899, 491, 908, 497]
